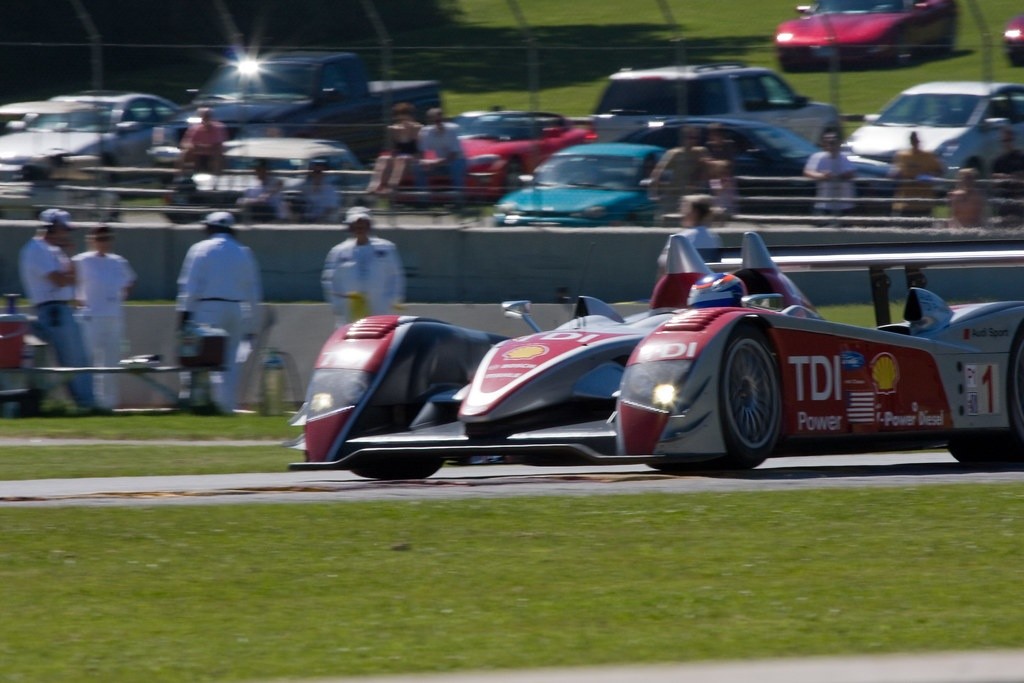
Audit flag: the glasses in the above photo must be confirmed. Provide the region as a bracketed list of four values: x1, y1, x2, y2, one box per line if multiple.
[94, 235, 113, 241]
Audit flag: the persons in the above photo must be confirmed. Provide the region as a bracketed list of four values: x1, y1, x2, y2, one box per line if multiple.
[174, 212, 261, 415]
[364, 104, 466, 198]
[657, 195, 724, 274]
[647, 123, 746, 216]
[236, 159, 342, 223]
[21, 209, 140, 415]
[178, 108, 226, 176]
[802, 127, 1024, 225]
[325, 206, 405, 325]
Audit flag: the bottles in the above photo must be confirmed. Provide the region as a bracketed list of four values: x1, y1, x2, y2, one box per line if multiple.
[259, 354, 288, 417]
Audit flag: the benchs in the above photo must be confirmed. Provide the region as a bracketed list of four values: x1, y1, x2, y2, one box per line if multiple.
[34, 364, 255, 415]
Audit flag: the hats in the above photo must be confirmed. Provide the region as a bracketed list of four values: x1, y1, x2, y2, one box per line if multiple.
[91, 225, 111, 234]
[342, 206, 373, 224]
[38, 208, 76, 229]
[200, 212, 235, 227]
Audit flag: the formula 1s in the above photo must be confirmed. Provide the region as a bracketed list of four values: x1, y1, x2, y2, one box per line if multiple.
[277, 230, 1024, 480]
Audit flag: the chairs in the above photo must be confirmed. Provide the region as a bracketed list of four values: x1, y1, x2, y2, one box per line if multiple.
[648, 233, 816, 318]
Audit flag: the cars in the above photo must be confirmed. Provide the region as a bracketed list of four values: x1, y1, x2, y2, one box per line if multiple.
[999, 15, 1024, 67]
[2, 52, 1024, 223]
[772, 0, 958, 67]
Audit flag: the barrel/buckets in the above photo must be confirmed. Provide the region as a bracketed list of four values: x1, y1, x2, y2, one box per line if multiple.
[0, 313, 30, 369]
